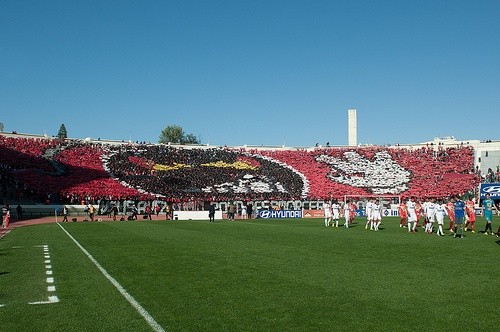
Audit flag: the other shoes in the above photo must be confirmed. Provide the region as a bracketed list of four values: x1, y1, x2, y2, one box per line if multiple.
[365, 225, 380, 232]
[400, 224, 499, 239]
[325, 222, 352, 228]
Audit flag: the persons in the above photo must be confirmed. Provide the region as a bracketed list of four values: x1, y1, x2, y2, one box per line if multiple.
[1, 127, 500, 241]
